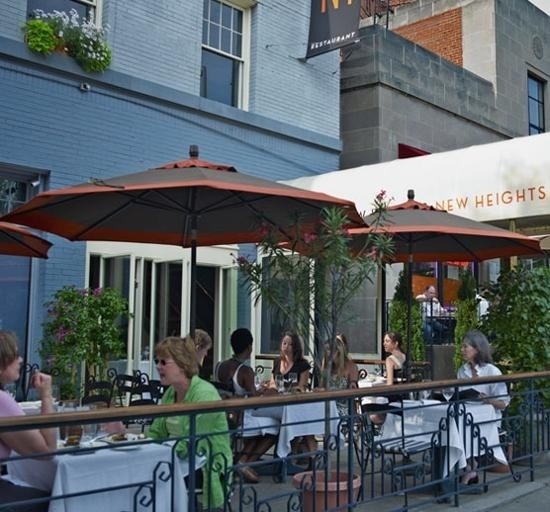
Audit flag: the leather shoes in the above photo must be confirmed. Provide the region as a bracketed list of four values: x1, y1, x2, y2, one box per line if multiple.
[236, 461, 259, 482]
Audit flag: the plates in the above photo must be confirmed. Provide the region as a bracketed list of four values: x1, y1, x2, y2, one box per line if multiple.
[103, 432, 152, 444]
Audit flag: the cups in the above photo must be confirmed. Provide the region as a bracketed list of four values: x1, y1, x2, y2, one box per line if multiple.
[61, 401, 76, 413]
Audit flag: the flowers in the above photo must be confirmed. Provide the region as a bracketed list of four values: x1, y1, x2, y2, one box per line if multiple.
[19, 7, 112, 75]
[33, 284, 136, 400]
[227, 189, 395, 482]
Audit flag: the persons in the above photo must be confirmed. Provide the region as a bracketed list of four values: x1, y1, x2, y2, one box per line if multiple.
[269, 331, 311, 393]
[211, 328, 281, 483]
[366, 332, 409, 425]
[144, 327, 235, 511]
[293, 337, 360, 466]
[454, 327, 511, 483]
[191, 329, 214, 368]
[415, 284, 449, 344]
[0, 329, 57, 512]
[336, 332, 349, 355]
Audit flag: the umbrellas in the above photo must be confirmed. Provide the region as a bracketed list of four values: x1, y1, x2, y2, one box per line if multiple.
[270, 188, 543, 370]
[0, 144, 371, 332]
[1, 222, 53, 261]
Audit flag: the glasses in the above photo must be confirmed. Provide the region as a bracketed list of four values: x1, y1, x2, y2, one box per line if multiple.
[154, 359, 176, 365]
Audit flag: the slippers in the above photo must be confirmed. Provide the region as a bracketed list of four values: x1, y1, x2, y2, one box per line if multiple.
[461, 475, 478, 485]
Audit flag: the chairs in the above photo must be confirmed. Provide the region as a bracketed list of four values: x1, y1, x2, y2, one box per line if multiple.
[355, 391, 442, 500]
[209, 378, 236, 445]
[77, 366, 163, 438]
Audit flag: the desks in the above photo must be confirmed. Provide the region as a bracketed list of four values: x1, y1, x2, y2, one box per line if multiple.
[365, 400, 494, 498]
[238, 390, 342, 481]
[0, 437, 189, 512]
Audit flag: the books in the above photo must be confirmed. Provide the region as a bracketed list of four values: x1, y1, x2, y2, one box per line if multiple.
[432, 387, 482, 404]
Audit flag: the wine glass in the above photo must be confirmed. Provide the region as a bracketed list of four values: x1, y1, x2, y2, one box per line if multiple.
[409, 379, 455, 402]
[375, 368, 381, 375]
[255, 373, 297, 393]
